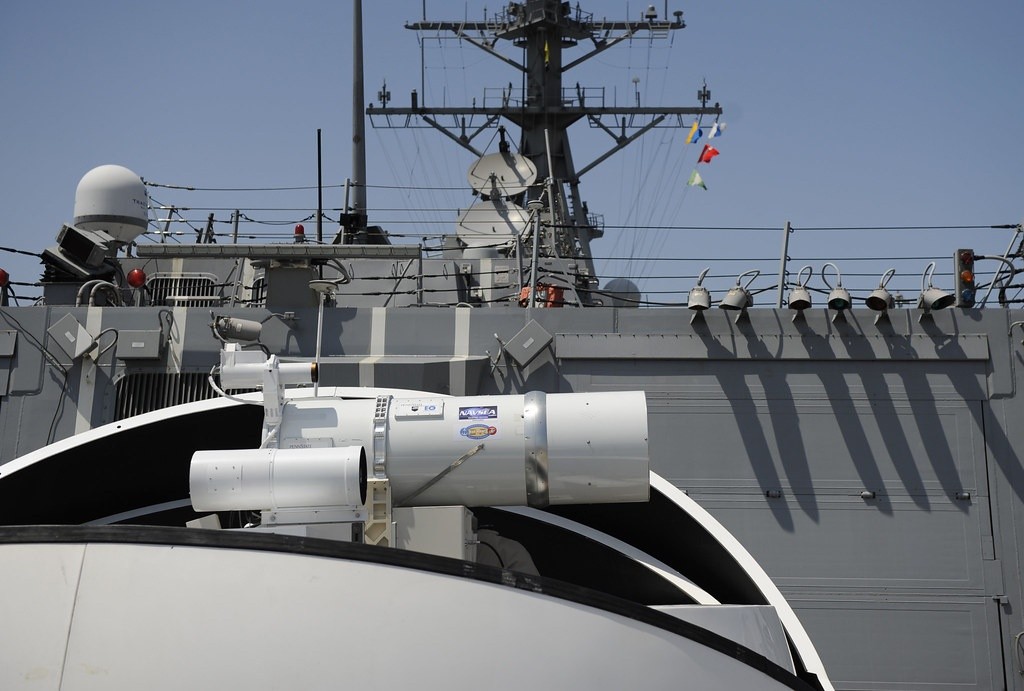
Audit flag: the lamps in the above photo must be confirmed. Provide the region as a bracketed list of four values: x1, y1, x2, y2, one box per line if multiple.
[719, 269, 761, 323]
[787, 265, 813, 321]
[822, 263, 852, 323]
[866, 268, 896, 325]
[687, 267, 711, 323]
[917, 261, 957, 322]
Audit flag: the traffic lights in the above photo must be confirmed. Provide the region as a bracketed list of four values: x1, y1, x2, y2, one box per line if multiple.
[956, 249, 975, 307]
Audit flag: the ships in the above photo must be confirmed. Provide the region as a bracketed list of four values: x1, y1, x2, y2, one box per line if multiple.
[0, 0, 1024, 691]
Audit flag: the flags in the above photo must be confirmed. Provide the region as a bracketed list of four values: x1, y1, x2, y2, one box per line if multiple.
[687, 170, 707, 190]
[698, 145, 719, 163]
[709, 123, 721, 138]
[685, 121, 702, 144]
